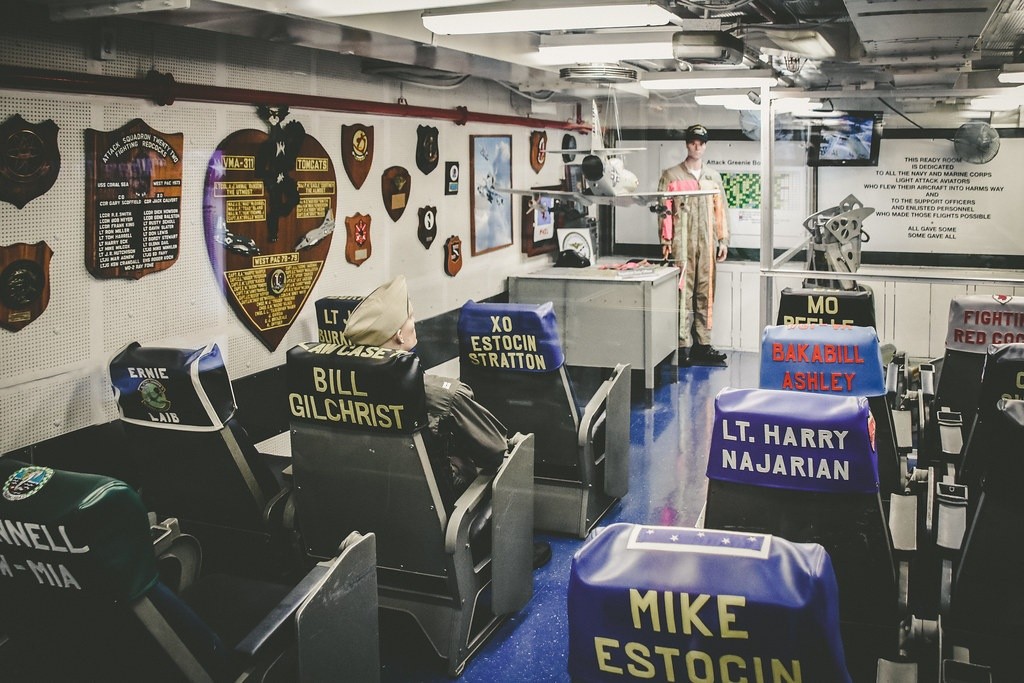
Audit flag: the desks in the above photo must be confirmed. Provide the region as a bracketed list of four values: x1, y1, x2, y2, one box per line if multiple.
[508, 264, 680, 409]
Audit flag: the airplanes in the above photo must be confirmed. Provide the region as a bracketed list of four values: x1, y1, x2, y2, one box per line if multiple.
[494, 98, 721, 214]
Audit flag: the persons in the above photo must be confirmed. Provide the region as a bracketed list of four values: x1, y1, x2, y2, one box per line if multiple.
[342, 274, 553, 578]
[658, 125, 730, 367]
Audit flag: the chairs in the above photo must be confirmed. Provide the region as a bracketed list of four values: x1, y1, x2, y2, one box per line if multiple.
[0, 283, 1024, 683]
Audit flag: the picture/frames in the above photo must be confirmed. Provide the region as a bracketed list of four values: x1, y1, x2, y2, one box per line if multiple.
[469, 135, 513, 256]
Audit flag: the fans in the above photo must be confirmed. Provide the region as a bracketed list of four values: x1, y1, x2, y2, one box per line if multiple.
[739, 110, 783, 142]
[955, 121, 1000, 164]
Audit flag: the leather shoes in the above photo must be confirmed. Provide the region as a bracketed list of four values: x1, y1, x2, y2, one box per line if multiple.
[533, 540, 551, 568]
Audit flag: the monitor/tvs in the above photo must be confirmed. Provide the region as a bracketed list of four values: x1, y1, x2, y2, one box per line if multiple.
[807, 109, 884, 167]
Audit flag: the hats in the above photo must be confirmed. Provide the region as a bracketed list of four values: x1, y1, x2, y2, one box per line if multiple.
[342, 275, 414, 346]
[685, 125, 710, 143]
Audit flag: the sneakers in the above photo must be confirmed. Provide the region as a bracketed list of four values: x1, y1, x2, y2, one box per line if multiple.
[679, 350, 691, 367]
[689, 345, 727, 362]
